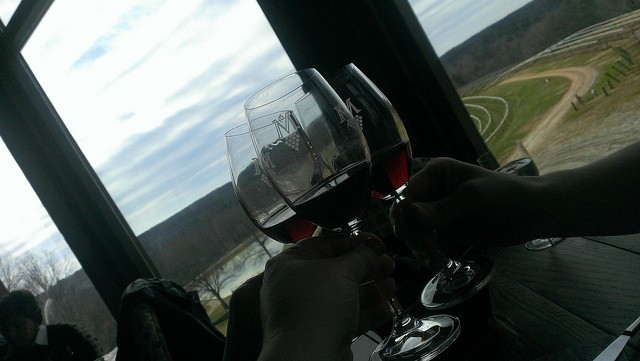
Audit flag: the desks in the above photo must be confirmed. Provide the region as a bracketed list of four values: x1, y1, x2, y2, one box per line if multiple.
[349, 235, 640, 360]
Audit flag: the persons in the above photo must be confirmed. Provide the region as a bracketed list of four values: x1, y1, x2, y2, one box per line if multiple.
[0, 289, 104, 361]
[257, 228, 387, 361]
[387, 140, 639, 361]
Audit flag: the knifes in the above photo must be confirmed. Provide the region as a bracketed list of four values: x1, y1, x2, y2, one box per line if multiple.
[592, 315, 640, 361]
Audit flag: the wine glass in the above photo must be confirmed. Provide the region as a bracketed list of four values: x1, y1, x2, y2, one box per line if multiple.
[477, 139, 564, 251]
[258, 66, 462, 361]
[293, 60, 497, 312]
[224, 108, 321, 244]
